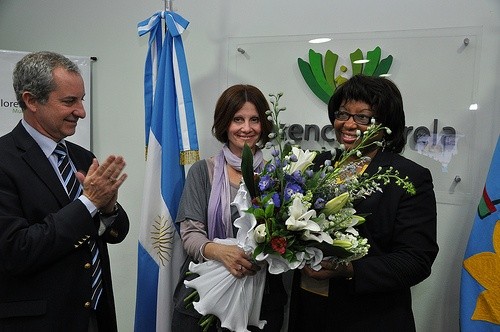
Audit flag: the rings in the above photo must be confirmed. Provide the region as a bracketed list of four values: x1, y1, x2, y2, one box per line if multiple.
[238, 265, 242, 271]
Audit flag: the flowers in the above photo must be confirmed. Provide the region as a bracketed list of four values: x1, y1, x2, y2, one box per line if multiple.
[183, 92, 416, 332]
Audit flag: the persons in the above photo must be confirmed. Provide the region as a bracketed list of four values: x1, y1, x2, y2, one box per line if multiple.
[171, 84, 285, 332]
[0, 52, 130, 332]
[288, 74, 439, 332]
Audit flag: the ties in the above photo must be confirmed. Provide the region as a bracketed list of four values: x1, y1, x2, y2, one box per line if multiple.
[53, 143, 103, 310]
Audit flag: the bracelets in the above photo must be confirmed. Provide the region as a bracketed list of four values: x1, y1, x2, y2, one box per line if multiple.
[98, 203, 118, 217]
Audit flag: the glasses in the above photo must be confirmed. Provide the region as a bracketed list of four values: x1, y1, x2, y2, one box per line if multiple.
[334, 111, 374, 125]
